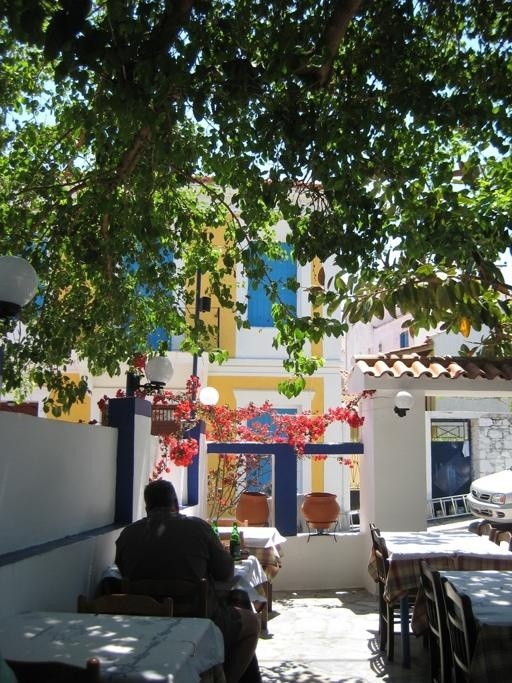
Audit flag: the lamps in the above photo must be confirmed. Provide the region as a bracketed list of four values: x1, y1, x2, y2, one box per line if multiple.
[0, 256, 38, 317]
[133, 356, 174, 391]
[393, 391, 415, 417]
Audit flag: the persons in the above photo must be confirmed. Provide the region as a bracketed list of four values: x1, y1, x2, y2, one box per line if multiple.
[114, 480, 261, 683]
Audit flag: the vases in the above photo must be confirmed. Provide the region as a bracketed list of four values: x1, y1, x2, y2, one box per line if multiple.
[300, 492, 340, 529]
[235, 492, 269, 527]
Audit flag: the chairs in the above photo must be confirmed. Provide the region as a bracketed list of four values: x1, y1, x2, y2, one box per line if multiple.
[4, 653, 102, 683]
[76, 591, 178, 619]
[214, 536, 287, 644]
[364, 523, 429, 668]
[103, 573, 208, 623]
[416, 559, 454, 682]
[440, 577, 499, 683]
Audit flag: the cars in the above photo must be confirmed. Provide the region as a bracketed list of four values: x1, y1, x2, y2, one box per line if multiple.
[467, 465, 512, 524]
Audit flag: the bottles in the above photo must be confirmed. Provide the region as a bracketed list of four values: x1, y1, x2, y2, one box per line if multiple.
[211, 519, 218, 539]
[230, 522, 241, 563]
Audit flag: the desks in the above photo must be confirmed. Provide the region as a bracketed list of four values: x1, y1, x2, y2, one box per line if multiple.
[367, 525, 512, 667]
[438, 565, 510, 683]
[2, 609, 227, 683]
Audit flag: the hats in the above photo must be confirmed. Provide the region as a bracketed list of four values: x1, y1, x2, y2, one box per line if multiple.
[145, 479, 175, 517]
